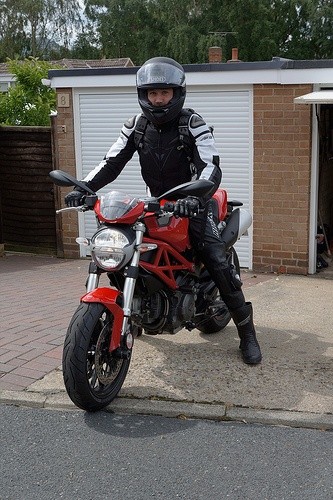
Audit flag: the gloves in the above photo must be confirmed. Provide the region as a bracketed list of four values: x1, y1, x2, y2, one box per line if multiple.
[173, 197, 199, 219]
[65, 191, 84, 206]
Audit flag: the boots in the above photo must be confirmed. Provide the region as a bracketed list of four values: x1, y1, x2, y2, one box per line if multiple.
[228, 302, 262, 364]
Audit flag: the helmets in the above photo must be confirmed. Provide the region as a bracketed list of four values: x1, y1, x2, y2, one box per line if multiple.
[135, 57, 186, 125]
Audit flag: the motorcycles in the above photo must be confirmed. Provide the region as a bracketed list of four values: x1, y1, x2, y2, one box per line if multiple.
[49, 169, 253, 412]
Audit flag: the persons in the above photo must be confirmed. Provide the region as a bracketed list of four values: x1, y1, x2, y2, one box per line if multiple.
[62, 55, 263, 365]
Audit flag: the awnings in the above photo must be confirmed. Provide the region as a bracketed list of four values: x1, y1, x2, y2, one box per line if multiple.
[293, 90, 333, 121]
